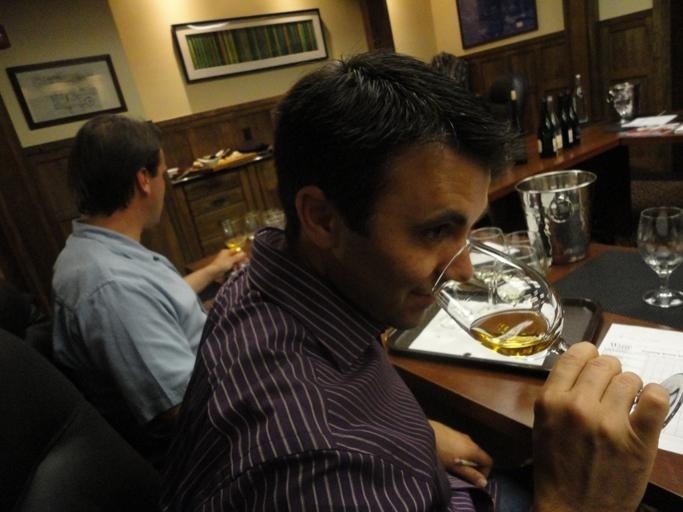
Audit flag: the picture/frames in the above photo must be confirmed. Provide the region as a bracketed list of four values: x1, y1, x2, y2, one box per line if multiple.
[170, 6, 329, 84]
[4, 53, 129, 130]
[455, 0, 540, 52]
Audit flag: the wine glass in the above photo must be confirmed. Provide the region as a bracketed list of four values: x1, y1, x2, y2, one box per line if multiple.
[432, 238, 683, 429]
[471, 227, 553, 279]
[614, 96, 632, 127]
[635, 207, 683, 308]
[220, 209, 286, 265]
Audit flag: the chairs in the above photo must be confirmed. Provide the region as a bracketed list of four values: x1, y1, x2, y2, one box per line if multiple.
[1, 327, 164, 512]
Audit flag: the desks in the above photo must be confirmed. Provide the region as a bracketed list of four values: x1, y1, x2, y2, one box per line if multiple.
[185, 228, 682, 503]
[489, 121, 620, 242]
[182, 116, 683, 512]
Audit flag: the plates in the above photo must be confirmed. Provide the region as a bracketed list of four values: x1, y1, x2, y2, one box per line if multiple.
[385, 286, 600, 379]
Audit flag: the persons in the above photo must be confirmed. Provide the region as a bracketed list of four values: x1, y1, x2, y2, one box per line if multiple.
[154, 47, 673, 512]
[49, 114, 248, 426]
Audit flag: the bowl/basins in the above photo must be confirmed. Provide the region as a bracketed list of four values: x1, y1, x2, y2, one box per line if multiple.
[166, 167, 179, 177]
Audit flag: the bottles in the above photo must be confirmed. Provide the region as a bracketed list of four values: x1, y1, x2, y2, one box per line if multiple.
[536, 88, 581, 158]
[573, 73, 591, 128]
[508, 89, 531, 167]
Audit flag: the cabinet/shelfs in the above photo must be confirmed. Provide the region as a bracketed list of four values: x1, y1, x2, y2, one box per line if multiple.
[623, 122, 683, 216]
[171, 150, 276, 259]
